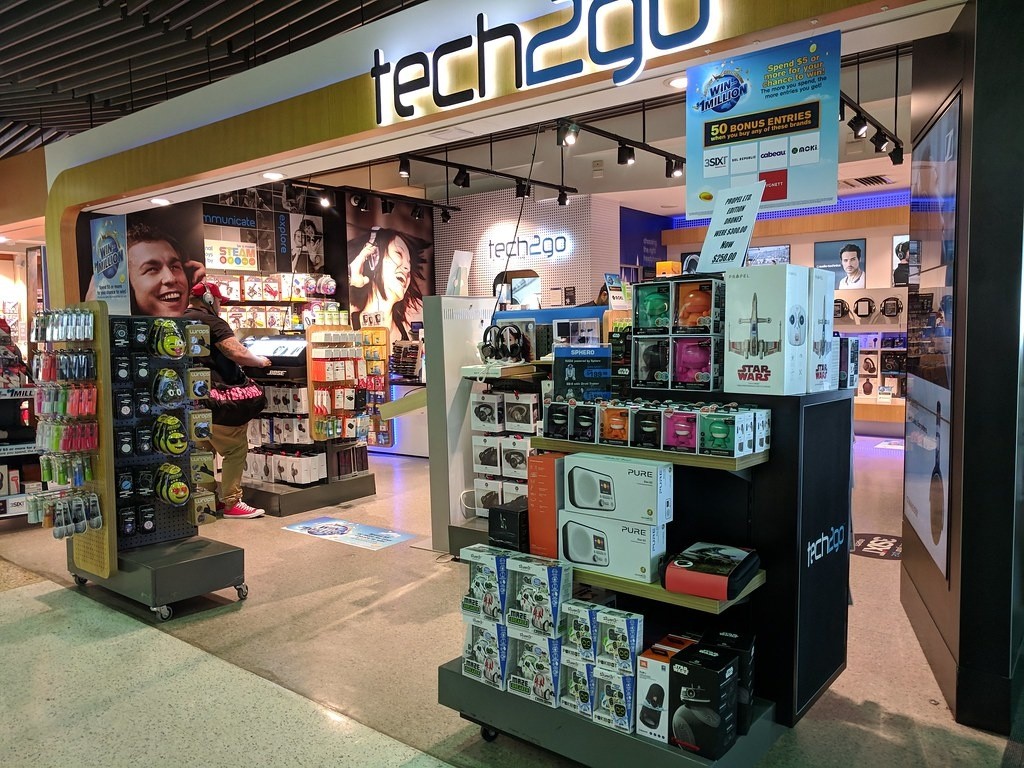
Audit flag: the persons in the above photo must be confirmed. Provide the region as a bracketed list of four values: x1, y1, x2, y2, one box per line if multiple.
[576, 282, 608, 307]
[292, 219, 323, 273]
[85, 223, 206, 318]
[347, 226, 423, 356]
[501, 324, 531, 361]
[181, 281, 272, 519]
[838, 243, 864, 289]
[893, 241, 909, 287]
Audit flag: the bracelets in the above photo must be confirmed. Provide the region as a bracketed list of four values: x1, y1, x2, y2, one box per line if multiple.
[348, 264, 351, 284]
[313, 263, 318, 266]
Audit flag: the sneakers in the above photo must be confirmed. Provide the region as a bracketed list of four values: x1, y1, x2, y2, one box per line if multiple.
[222, 497, 265, 519]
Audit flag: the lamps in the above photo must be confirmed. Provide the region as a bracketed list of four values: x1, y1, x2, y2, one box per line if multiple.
[348, 47, 911, 225]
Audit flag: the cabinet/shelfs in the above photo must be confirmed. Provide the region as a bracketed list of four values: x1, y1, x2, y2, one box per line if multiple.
[0, 386, 44, 519]
[527, 439, 771, 616]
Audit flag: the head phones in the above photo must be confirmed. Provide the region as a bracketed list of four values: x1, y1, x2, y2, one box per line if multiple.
[365, 226, 382, 271]
[854, 298, 876, 315]
[197, 281, 214, 306]
[880, 297, 904, 315]
[863, 358, 876, 373]
[474, 404, 528, 510]
[898, 245, 905, 260]
[482, 324, 523, 360]
[834, 299, 850, 317]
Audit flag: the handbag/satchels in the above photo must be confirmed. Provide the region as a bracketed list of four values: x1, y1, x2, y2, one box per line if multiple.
[204, 369, 267, 426]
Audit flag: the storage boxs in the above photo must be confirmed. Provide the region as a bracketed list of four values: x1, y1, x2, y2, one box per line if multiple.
[187, 274, 395, 527]
[459, 264, 909, 760]
[0, 465, 42, 497]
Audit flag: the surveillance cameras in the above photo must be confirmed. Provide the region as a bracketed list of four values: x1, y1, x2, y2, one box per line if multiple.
[350, 195, 361, 206]
